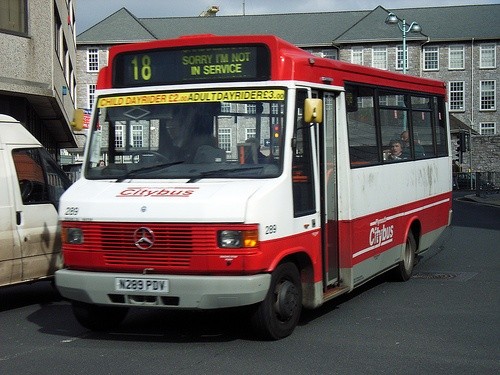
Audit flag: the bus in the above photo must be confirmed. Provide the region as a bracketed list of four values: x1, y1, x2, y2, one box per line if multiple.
[54, 34, 453, 341]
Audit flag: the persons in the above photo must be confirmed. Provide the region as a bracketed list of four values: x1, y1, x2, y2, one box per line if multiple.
[97, 160, 106, 168]
[383, 130, 426, 162]
[154, 117, 201, 164]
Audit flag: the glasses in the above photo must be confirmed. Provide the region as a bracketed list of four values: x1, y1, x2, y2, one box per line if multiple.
[165, 125, 176, 129]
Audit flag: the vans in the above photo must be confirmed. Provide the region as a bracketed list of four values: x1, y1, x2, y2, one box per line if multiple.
[0, 113, 73, 288]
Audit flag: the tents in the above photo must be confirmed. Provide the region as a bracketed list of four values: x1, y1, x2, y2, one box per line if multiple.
[292, 102, 480, 191]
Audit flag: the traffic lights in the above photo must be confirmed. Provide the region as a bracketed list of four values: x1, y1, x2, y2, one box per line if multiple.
[270, 124, 281, 146]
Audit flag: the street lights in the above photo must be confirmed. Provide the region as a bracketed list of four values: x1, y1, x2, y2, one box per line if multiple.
[385, 12, 423, 74]
